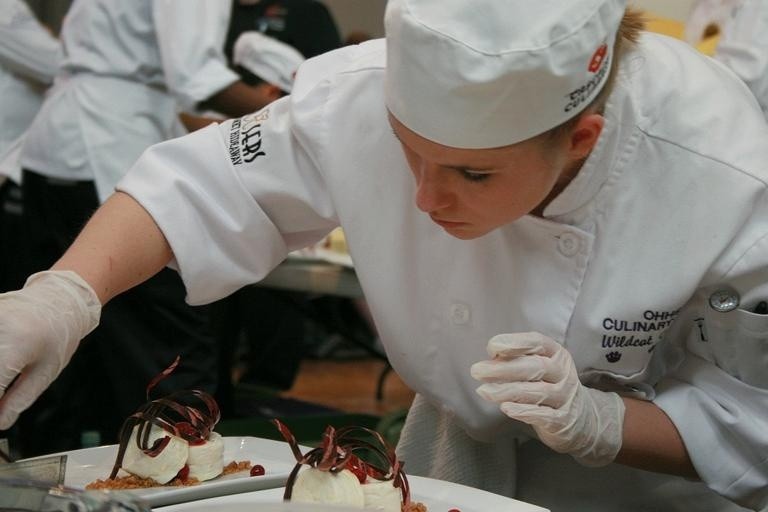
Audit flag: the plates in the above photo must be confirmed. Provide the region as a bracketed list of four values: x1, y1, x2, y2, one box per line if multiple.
[15, 435, 314, 508]
[149, 474, 550, 512]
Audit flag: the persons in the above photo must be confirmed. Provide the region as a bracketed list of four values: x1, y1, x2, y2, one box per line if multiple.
[0, 1, 766, 512]
[1, 2, 343, 453]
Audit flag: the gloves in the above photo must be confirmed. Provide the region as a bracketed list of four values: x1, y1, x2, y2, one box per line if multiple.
[0, 267, 103, 434]
[469, 331, 627, 469]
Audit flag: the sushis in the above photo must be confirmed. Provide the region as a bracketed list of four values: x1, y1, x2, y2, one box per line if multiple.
[189, 433, 224, 482]
[292, 468, 365, 510]
[357, 477, 403, 512]
[121, 421, 188, 485]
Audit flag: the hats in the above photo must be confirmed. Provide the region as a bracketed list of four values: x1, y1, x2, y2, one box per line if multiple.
[382, 0, 628, 151]
[232, 31, 307, 95]
